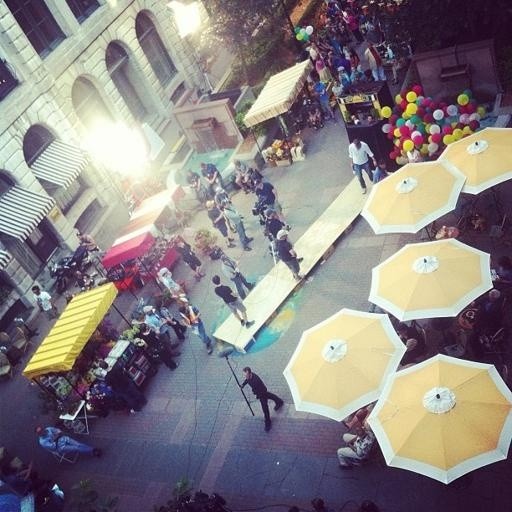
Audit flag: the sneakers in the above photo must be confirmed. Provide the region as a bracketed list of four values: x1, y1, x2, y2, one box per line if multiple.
[273, 400, 284, 411]
[226, 235, 237, 249]
[240, 236, 255, 252]
[264, 422, 272, 432]
[206, 344, 212, 355]
[361, 186, 366, 194]
[240, 318, 256, 329]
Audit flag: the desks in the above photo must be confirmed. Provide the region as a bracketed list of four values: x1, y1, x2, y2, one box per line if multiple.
[58, 399, 97, 435]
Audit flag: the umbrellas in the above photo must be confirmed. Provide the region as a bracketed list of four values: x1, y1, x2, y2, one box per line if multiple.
[354, 158, 467, 235]
[436, 125, 511, 197]
[280, 306, 408, 424]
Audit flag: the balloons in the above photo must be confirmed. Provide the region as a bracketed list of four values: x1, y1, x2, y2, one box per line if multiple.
[380, 83, 486, 165]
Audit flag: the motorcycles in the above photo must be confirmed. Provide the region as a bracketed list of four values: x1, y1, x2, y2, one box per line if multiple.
[50, 245, 91, 293]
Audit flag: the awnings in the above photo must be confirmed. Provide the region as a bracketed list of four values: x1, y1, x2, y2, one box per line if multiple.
[33, 139, 90, 190]
[1, 186, 53, 240]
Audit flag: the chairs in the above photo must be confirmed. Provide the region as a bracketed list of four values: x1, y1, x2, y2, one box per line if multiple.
[1, 327, 37, 380]
[48, 450, 79, 465]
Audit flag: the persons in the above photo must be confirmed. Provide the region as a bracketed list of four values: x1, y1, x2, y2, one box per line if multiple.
[384, 146, 401, 178]
[31, 161, 302, 410]
[35, 425, 104, 459]
[337, 404, 377, 469]
[1, 459, 65, 504]
[385, 318, 427, 365]
[286, 0, 415, 132]
[348, 136, 377, 197]
[4, 446, 58, 492]
[433, 210, 510, 368]
[239, 364, 284, 433]
